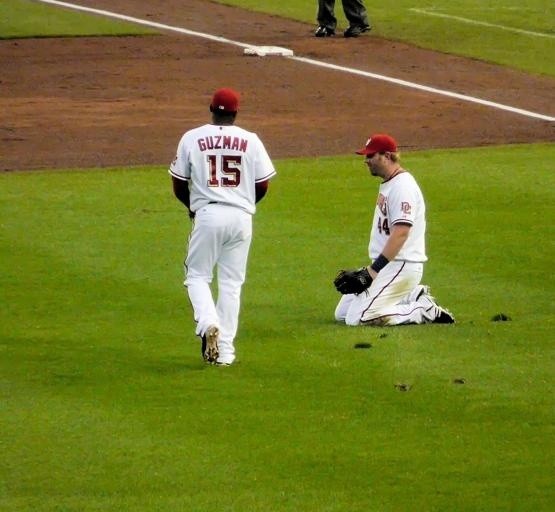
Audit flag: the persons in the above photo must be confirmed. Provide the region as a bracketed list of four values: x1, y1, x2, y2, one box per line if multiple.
[315, 0, 371, 38]
[332, 135, 456, 327]
[166, 89, 277, 369]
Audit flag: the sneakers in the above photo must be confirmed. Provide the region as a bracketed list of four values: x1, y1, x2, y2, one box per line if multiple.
[416, 286, 456, 324]
[315, 26, 335, 36]
[202, 326, 219, 366]
[344, 24, 371, 37]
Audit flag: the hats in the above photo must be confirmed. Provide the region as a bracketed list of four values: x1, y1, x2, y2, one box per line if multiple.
[212, 87, 240, 112]
[355, 134, 397, 155]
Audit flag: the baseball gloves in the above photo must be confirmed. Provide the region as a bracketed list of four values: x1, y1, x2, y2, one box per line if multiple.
[334, 266, 372, 295]
